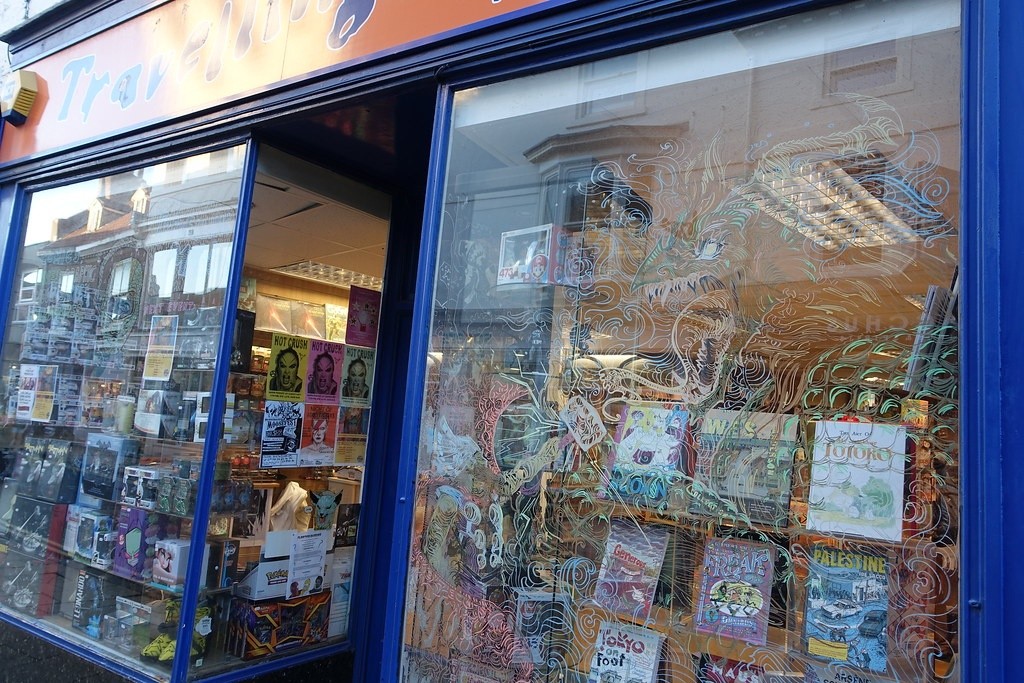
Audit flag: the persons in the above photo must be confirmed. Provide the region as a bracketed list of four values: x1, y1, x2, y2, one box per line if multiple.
[343, 409, 362, 433]
[300, 418, 333, 461]
[510, 233, 543, 280]
[289, 575, 323, 598]
[269, 347, 370, 399]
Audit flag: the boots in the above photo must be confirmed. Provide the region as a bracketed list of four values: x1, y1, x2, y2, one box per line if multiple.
[159, 606, 210, 662]
[140, 599, 182, 657]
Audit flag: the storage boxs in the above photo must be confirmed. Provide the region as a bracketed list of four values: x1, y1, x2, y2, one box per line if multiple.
[0, 433, 204, 653]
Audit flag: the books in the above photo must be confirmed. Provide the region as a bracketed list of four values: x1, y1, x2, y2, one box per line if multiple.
[397, 399, 907, 683]
[19, 282, 130, 368]
[0, 368, 154, 436]
[902, 265, 959, 397]
